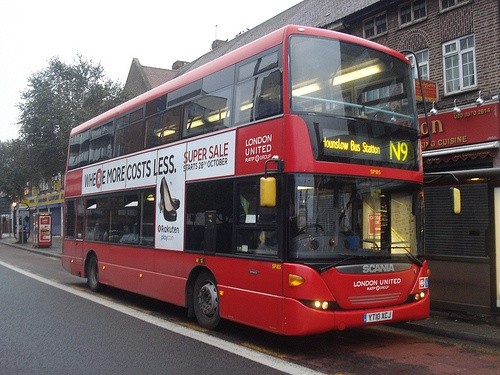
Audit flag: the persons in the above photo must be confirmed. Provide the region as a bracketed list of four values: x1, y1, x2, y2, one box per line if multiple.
[223, 183, 249, 217]
[118, 225, 140, 244]
[87, 220, 106, 240]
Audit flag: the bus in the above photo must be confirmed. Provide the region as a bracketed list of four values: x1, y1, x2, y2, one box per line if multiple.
[61, 23, 432, 338]
